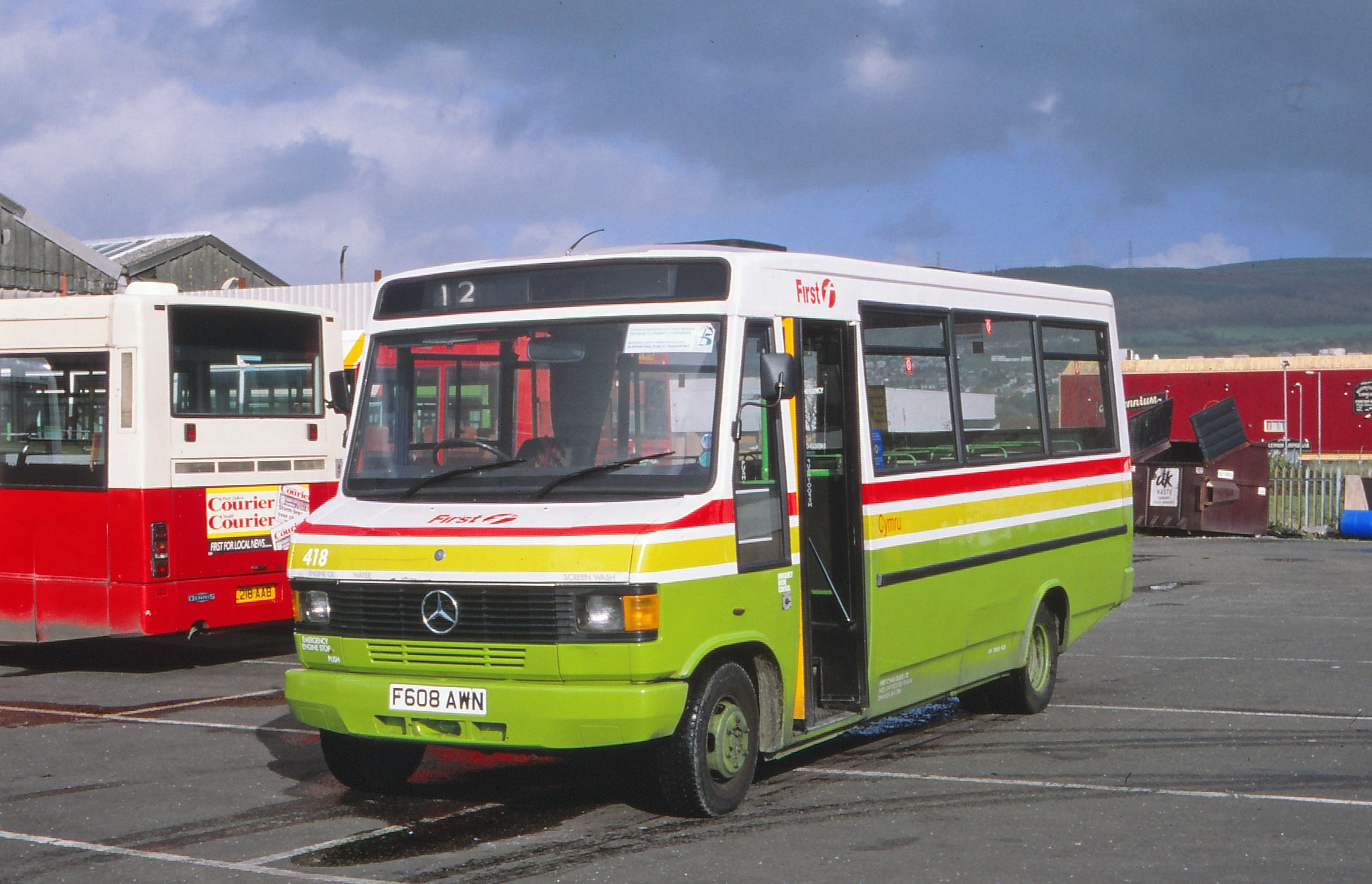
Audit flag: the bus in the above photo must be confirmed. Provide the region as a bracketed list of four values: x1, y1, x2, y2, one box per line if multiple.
[0, 279, 340, 663]
[282, 238, 1136, 819]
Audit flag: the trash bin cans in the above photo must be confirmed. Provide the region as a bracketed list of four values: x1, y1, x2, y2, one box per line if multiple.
[1127, 397, 1272, 536]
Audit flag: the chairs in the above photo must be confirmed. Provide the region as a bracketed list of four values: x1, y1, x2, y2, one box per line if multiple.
[740, 441, 1082, 475]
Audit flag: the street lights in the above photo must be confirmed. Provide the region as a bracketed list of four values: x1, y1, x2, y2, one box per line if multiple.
[1279, 360, 1322, 466]
